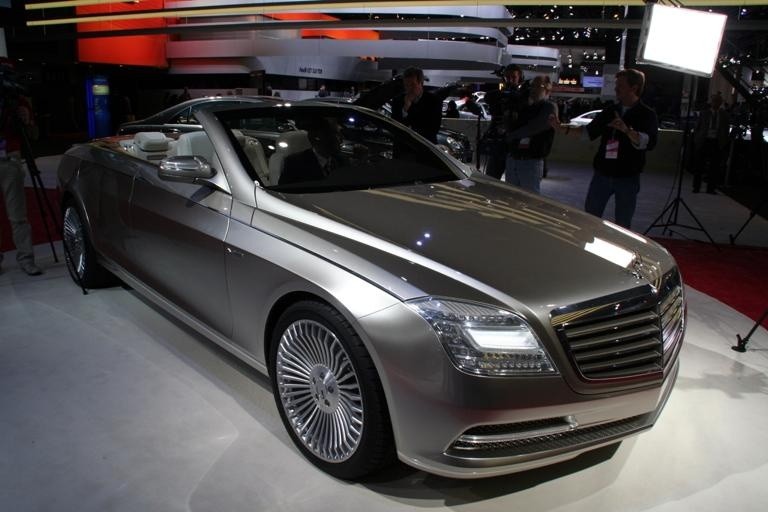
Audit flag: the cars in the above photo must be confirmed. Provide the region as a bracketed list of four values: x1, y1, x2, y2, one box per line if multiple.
[452, 88, 493, 120]
[55, 95, 687, 483]
[569, 106, 604, 127]
[305, 94, 474, 162]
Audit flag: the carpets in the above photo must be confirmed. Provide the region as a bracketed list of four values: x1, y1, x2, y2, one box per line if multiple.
[653, 236, 767, 334]
[1, 180, 66, 255]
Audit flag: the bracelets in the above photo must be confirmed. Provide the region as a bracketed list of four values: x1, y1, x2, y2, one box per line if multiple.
[564, 124, 571, 136]
[625, 124, 634, 135]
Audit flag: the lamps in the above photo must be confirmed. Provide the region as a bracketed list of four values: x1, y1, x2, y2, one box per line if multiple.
[637, 4, 729, 243]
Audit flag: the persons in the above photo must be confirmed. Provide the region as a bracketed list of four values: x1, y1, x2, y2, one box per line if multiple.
[692, 94, 729, 195]
[502, 76, 558, 195]
[499, 68, 536, 149]
[341, 88, 352, 97]
[287, 116, 350, 182]
[393, 67, 508, 179]
[158, 86, 191, 109]
[264, 82, 272, 96]
[318, 85, 330, 97]
[120, 96, 136, 122]
[554, 98, 616, 123]
[0, 56, 43, 276]
[350, 86, 358, 96]
[543, 78, 561, 178]
[547, 70, 659, 233]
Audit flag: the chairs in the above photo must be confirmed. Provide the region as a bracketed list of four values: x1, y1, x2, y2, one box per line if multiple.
[129, 127, 312, 188]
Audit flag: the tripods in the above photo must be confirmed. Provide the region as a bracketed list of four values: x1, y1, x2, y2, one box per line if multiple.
[10, 106, 88, 295]
[642, 75, 716, 247]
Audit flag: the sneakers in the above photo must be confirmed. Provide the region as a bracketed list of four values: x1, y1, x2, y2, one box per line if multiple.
[20, 262, 41, 275]
